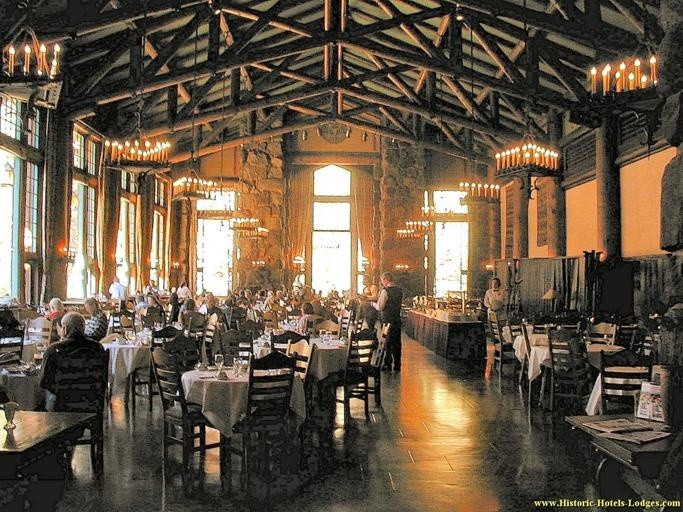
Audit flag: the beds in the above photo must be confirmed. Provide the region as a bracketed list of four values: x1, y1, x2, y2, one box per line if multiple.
[532, 341, 627, 402]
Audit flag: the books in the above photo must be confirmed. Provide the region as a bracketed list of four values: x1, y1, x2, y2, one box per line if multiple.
[581, 417, 671, 445]
[638, 364, 666, 421]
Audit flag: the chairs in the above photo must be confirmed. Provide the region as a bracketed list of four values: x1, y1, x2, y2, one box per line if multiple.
[519, 323, 549, 405]
[287, 339, 315, 385]
[345, 323, 391, 418]
[149, 326, 186, 412]
[269, 329, 310, 357]
[223, 352, 298, 488]
[587, 321, 617, 346]
[149, 350, 231, 494]
[618, 326, 637, 349]
[600, 349, 652, 415]
[301, 343, 338, 469]
[547, 327, 596, 411]
[54, 348, 109, 472]
[487, 310, 527, 376]
[218, 330, 254, 367]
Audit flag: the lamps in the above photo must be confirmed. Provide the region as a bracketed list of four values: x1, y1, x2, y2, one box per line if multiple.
[196, 29, 252, 219]
[460, 27, 501, 202]
[495, 0, 560, 172]
[0, 1, 63, 80]
[420, 69, 470, 223]
[104, 3, 172, 165]
[171, 26, 217, 200]
[231, 86, 261, 230]
[590, 16, 660, 94]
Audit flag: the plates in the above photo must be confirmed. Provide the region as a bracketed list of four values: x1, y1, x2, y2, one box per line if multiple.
[365, 297, 380, 303]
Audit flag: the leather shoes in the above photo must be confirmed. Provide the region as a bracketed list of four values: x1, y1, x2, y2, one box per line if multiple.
[394, 366, 400, 372]
[382, 365, 392, 371]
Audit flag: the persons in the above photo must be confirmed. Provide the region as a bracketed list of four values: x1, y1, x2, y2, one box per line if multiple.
[369, 272, 403, 371]
[80, 298, 107, 343]
[320, 291, 356, 334]
[134, 296, 147, 309]
[357, 273, 378, 319]
[226, 288, 255, 324]
[287, 290, 299, 317]
[179, 299, 204, 326]
[253, 289, 289, 317]
[38, 310, 109, 414]
[175, 281, 192, 299]
[206, 296, 229, 330]
[141, 279, 158, 296]
[297, 302, 321, 332]
[108, 276, 126, 309]
[297, 288, 322, 305]
[146, 295, 164, 317]
[44, 297, 65, 320]
[482, 277, 508, 345]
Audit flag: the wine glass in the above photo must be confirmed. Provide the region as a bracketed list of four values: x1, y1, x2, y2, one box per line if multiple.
[118, 330, 159, 346]
[215, 354, 225, 377]
[233, 357, 243, 379]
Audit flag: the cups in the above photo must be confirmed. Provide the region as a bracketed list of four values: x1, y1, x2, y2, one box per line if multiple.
[2, 400, 19, 435]
[319, 331, 340, 347]
[197, 362, 207, 372]
[258, 333, 270, 347]
